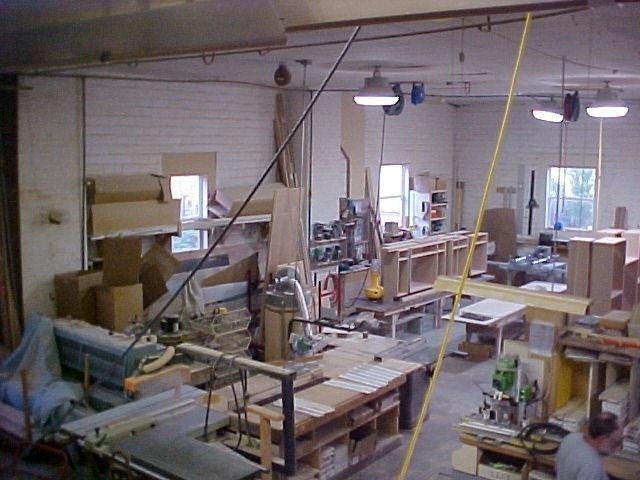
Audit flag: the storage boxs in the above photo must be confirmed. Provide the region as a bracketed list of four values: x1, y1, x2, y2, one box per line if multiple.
[50, 171, 287, 335]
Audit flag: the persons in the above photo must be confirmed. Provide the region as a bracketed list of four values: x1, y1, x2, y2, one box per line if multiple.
[555, 412, 624, 480]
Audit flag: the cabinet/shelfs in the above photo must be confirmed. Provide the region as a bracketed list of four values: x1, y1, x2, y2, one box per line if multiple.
[589, 237, 640, 314]
[238, 366, 409, 480]
[313, 236, 348, 267]
[380, 230, 489, 300]
[409, 189, 449, 235]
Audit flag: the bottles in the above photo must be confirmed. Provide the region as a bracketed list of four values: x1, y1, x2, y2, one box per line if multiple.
[365, 271, 384, 301]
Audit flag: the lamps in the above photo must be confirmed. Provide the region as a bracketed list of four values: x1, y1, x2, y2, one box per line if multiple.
[530, 80, 630, 125]
[353, 64, 402, 107]
